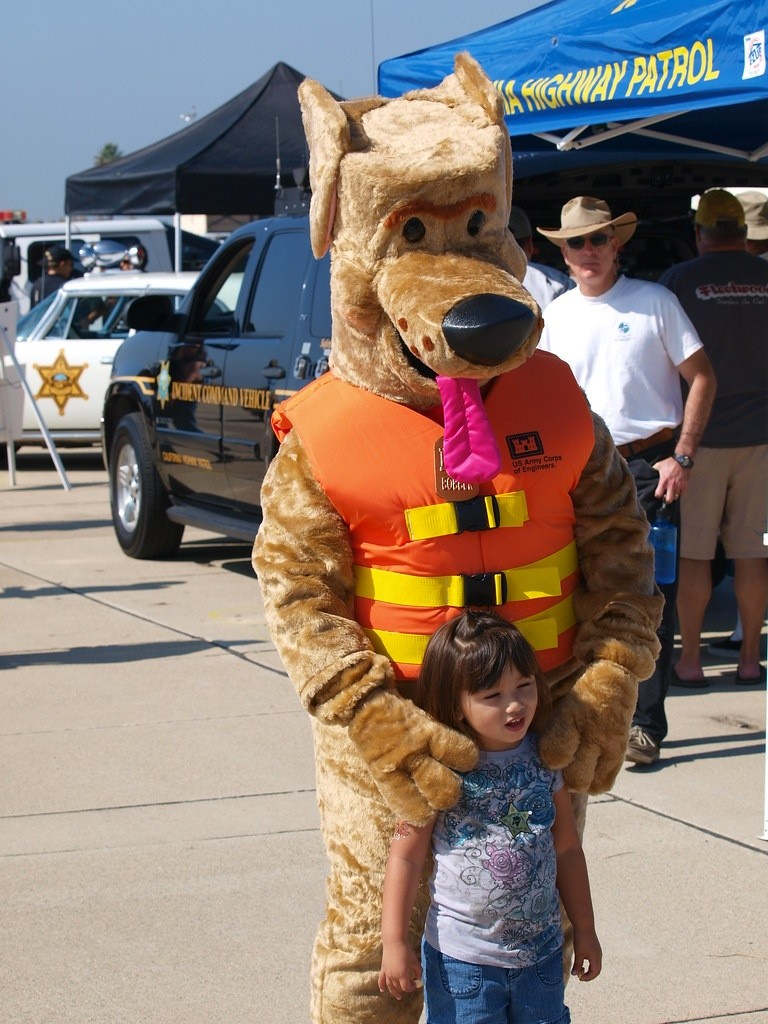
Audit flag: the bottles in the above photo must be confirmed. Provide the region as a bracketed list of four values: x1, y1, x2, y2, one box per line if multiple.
[649, 507, 679, 585]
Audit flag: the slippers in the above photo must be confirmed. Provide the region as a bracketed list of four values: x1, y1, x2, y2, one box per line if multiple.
[734, 662, 767, 684]
[670, 664, 708, 688]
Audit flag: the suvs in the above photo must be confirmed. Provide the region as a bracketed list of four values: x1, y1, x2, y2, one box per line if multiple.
[102, 216, 692, 560]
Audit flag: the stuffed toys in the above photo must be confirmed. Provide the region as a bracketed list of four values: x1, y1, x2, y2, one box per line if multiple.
[253, 49, 666, 1024]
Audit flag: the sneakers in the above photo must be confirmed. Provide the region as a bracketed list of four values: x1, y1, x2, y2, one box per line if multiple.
[626, 725, 660, 765]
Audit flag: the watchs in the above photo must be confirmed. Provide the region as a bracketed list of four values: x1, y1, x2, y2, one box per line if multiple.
[672, 453, 694, 468]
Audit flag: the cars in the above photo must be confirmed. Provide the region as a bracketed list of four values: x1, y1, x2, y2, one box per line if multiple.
[0, 272, 294, 467]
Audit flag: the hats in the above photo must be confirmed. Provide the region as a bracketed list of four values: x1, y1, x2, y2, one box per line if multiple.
[46, 246, 79, 263]
[696, 189, 746, 228]
[536, 196, 637, 248]
[734, 190, 768, 240]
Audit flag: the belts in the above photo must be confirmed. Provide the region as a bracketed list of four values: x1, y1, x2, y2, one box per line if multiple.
[616, 427, 675, 458]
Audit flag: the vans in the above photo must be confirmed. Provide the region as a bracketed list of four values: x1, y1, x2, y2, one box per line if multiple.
[0, 217, 231, 312]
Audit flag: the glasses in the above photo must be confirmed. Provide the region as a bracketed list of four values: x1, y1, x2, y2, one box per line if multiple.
[567, 233, 609, 248]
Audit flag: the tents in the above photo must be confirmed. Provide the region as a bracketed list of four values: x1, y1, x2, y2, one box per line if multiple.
[64, 60, 359, 311]
[376, 0, 768, 174]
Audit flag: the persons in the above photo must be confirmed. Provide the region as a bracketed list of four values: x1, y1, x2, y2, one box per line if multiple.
[658, 190, 768, 688]
[119, 243, 148, 270]
[538, 196, 717, 766]
[378, 608, 603, 1024]
[736, 192, 768, 264]
[30, 246, 105, 336]
[508, 205, 576, 311]
